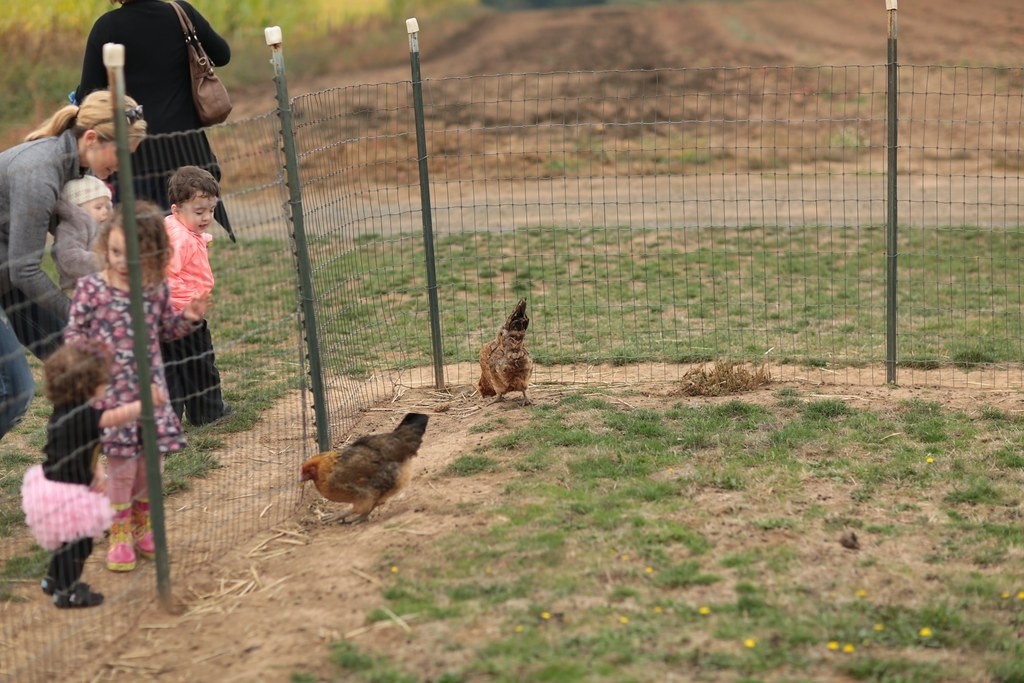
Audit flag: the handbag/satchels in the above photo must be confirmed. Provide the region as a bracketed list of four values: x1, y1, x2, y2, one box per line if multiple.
[168, 0, 231, 126]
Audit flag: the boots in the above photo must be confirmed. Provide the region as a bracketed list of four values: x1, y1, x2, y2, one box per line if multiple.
[106, 502, 136, 571]
[132, 497, 157, 560]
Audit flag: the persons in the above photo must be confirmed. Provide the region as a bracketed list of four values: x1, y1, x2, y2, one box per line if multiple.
[63, 195, 191, 573]
[76, 0, 236, 241]
[0, 89, 146, 443]
[157, 165, 232, 427]
[19, 334, 162, 608]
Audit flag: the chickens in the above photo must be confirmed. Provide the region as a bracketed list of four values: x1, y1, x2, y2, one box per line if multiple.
[300, 412, 430, 527]
[479, 297, 536, 406]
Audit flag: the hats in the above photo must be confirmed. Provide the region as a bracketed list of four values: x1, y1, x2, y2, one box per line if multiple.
[61, 174, 113, 208]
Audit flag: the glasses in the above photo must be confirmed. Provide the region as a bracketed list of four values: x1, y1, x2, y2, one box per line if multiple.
[89, 105, 143, 131]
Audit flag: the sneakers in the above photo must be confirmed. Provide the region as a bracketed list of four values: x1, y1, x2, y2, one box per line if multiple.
[41, 576, 103, 608]
